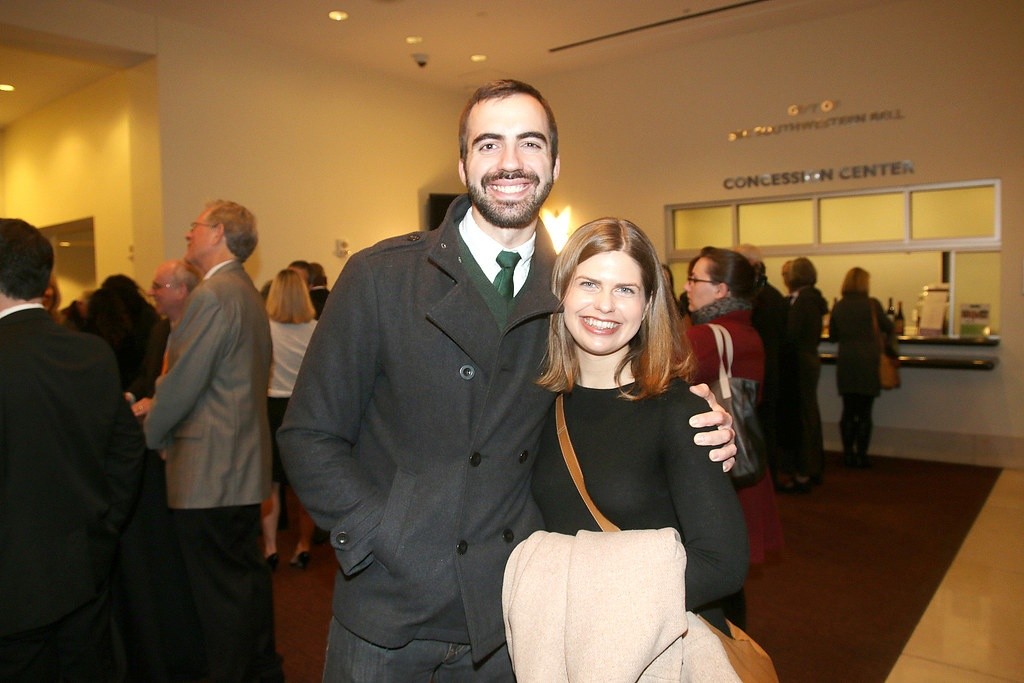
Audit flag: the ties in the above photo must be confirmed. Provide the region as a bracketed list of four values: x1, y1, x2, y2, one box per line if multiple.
[493, 250, 522, 302]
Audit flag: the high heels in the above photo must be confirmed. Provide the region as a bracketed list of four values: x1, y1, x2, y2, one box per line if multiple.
[773, 475, 811, 493]
[266, 553, 279, 571]
[811, 474, 823, 485]
[289, 551, 310, 571]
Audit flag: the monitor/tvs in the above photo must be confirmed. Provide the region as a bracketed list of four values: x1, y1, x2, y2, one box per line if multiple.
[429, 193, 460, 232]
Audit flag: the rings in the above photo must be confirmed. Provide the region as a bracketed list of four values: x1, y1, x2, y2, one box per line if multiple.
[723, 428, 733, 442]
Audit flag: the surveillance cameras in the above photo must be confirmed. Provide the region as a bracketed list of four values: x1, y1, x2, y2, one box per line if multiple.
[413, 53, 429, 67]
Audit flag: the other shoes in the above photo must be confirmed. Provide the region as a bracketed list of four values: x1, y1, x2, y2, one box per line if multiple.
[856, 454, 871, 467]
[845, 453, 856, 465]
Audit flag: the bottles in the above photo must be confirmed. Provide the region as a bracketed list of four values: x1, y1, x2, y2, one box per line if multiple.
[820, 308, 832, 338]
[887, 297, 904, 336]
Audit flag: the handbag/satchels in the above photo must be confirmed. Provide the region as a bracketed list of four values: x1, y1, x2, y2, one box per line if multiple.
[879, 351, 899, 389]
[703, 322, 765, 484]
[696, 615, 778, 683]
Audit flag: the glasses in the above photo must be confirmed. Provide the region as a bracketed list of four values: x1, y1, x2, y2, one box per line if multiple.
[688, 277, 730, 291]
[191, 223, 214, 229]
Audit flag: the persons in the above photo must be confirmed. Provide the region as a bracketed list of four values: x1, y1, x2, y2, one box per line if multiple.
[275, 78, 737, 683]
[259, 259, 330, 568]
[0, 218, 146, 683]
[65, 257, 205, 682]
[662, 246, 828, 566]
[142, 200, 285, 683]
[533, 215, 751, 635]
[828, 267, 886, 466]
